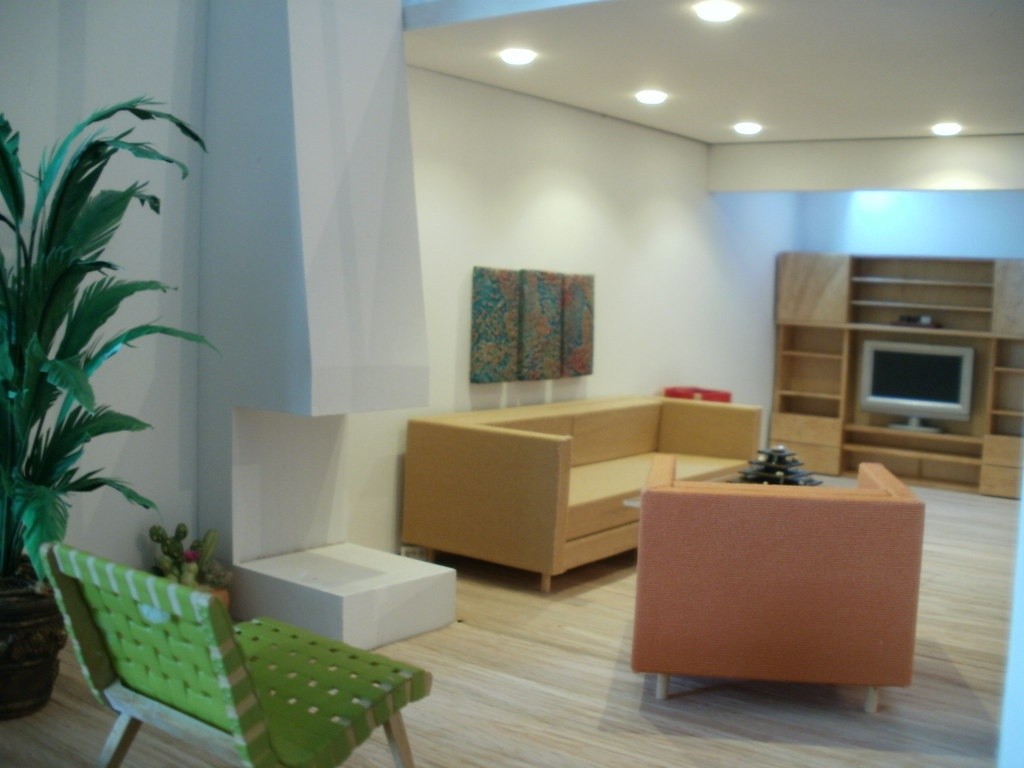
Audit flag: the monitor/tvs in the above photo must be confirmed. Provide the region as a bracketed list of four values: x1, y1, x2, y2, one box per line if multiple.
[859, 340, 974, 433]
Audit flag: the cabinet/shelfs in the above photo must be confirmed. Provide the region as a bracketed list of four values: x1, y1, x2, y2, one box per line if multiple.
[769, 252, 1024, 500]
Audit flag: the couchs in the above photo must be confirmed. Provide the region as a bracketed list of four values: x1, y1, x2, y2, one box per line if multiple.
[633, 462, 925, 714]
[403, 396, 761, 591]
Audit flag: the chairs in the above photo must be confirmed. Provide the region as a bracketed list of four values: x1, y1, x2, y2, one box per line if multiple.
[38, 542, 432, 768]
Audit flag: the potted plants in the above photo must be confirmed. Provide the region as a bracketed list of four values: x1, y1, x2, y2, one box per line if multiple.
[0, 96, 214, 719]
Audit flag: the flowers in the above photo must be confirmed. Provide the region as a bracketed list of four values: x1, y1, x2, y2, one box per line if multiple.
[146, 526, 220, 591]
